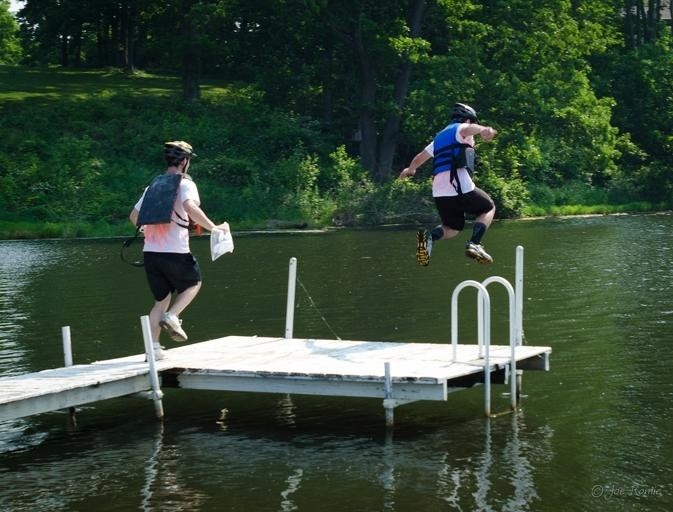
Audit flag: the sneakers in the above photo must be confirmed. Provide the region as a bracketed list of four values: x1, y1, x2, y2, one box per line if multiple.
[466, 240, 493, 263]
[160, 312, 188, 342]
[416, 229, 433, 265]
[144, 347, 167, 363]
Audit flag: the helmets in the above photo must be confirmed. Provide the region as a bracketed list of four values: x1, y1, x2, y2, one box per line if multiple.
[451, 103, 478, 124]
[165, 140, 199, 160]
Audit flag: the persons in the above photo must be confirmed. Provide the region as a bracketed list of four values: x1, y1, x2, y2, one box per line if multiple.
[400, 103, 496, 266]
[129, 141, 228, 361]
